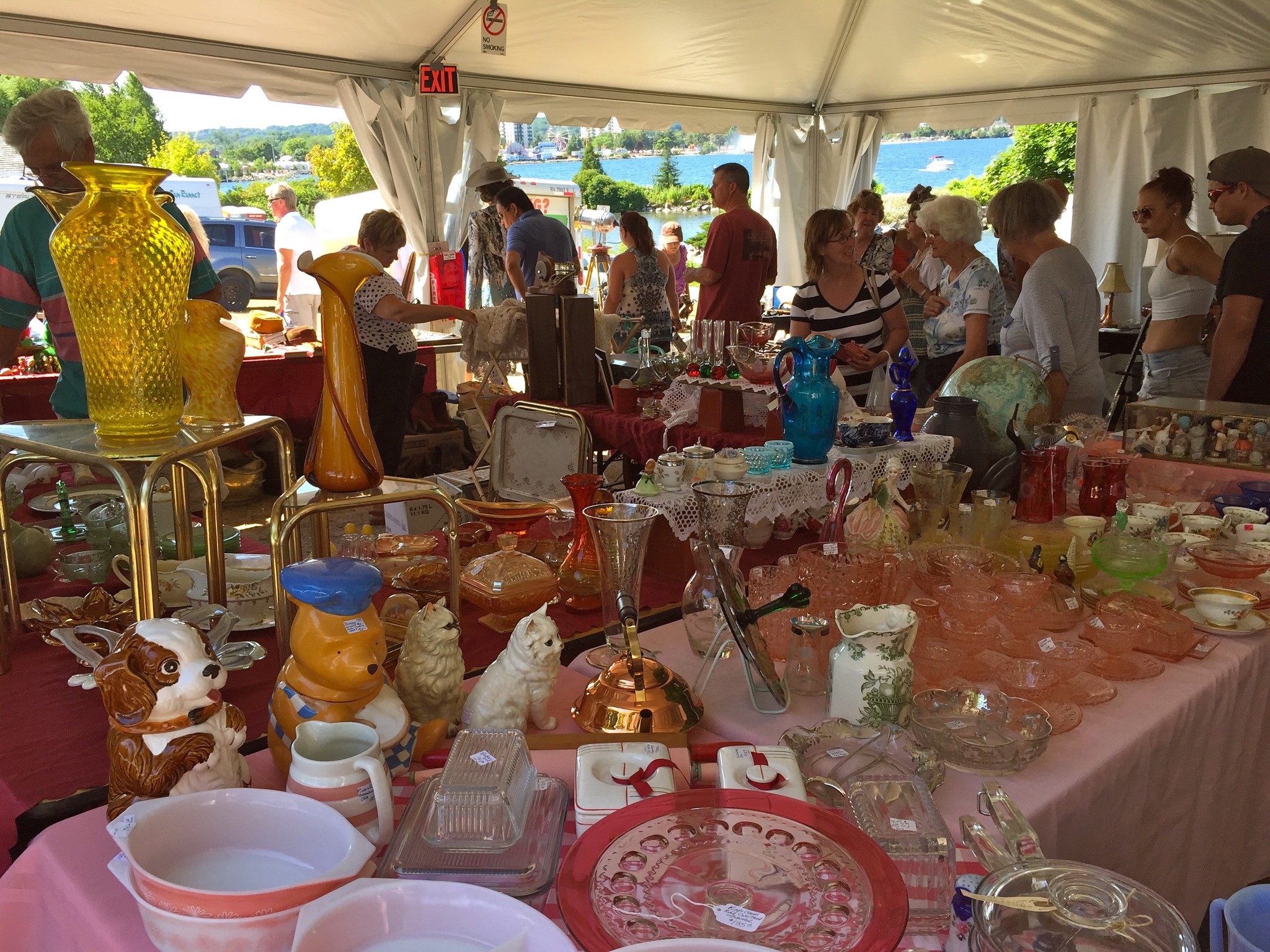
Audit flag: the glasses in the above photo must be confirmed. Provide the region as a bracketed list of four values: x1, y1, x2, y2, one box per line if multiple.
[923, 230, 941, 240]
[22, 143, 77, 180]
[1208, 183, 1236, 202]
[1132, 204, 1175, 222]
[823, 230, 858, 244]
[498, 205, 511, 219]
[269, 197, 284, 203]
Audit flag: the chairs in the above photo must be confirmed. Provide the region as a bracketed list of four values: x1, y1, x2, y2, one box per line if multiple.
[262, 236, 274, 248]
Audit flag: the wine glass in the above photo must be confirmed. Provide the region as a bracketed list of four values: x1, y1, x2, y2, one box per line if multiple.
[582, 503, 659, 669]
[636, 385, 672, 421]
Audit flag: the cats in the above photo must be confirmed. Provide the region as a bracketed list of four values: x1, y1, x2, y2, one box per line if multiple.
[393, 595, 467, 741]
[459, 599, 566, 735]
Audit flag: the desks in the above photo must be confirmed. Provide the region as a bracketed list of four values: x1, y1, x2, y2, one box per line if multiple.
[570, 569, 1270, 936]
[1099, 323, 1149, 355]
[0, 461, 815, 880]
[489, 394, 782, 490]
[1080, 432, 1270, 502]
[0, 331, 463, 454]
[0, 665, 1106, 952]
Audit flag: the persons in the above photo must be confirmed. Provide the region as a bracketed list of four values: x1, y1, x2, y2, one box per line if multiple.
[916, 195, 1005, 409]
[1131, 167, 1224, 430]
[987, 181, 1106, 422]
[656, 221, 693, 314]
[340, 209, 478, 526]
[465, 162, 520, 383]
[790, 209, 910, 408]
[683, 163, 778, 354]
[0, 88, 222, 419]
[1203, 146, 1270, 405]
[494, 187, 581, 303]
[602, 211, 682, 353]
[883, 185, 948, 357]
[176, 204, 210, 260]
[847, 189, 894, 275]
[997, 178, 1069, 304]
[266, 182, 321, 332]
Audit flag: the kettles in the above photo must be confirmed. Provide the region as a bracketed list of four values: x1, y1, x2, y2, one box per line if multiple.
[570, 589, 704, 733]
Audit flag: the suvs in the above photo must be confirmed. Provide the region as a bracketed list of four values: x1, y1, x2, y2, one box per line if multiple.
[199, 218, 277, 312]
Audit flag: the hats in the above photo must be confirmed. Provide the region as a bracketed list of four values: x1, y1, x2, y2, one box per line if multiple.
[1207, 146, 1270, 198]
[661, 221, 683, 243]
[465, 162, 520, 188]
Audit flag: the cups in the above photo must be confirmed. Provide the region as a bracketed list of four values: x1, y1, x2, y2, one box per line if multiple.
[1063, 503, 1270, 584]
[51, 496, 160, 584]
[737, 440, 794, 474]
[837, 416, 893, 448]
[650, 351, 692, 382]
[1209, 884, 1270, 952]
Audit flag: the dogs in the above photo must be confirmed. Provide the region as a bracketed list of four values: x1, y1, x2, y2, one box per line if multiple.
[92, 616, 253, 825]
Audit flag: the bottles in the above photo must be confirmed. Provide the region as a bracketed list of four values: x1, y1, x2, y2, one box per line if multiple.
[341, 524, 377, 562]
[629, 329, 666, 386]
[25, 161, 385, 494]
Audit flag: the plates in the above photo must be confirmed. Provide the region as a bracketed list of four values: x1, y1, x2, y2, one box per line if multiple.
[777, 717, 946, 806]
[28, 483, 140, 512]
[159, 526, 241, 561]
[378, 534, 571, 597]
[114, 587, 275, 631]
[1173, 602, 1270, 636]
[918, 628, 1165, 736]
[945, 873, 981, 952]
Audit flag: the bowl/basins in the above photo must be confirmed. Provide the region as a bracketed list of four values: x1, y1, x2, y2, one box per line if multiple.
[910, 686, 1053, 775]
[1187, 587, 1260, 630]
[107, 788, 577, 951]
[905, 544, 1020, 600]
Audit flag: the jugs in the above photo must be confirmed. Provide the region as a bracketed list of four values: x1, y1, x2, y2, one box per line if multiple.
[783, 612, 830, 697]
[824, 603, 919, 730]
[111, 548, 274, 625]
[681, 538, 746, 660]
[286, 720, 394, 861]
[452, 497, 564, 535]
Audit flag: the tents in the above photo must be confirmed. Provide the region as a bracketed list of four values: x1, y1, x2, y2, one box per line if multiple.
[0, 0, 1270, 301]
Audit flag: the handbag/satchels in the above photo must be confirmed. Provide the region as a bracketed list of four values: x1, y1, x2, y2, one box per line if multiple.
[865, 350, 900, 408]
[864, 266, 919, 371]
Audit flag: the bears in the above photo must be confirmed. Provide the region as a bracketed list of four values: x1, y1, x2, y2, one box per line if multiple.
[264, 592, 450, 780]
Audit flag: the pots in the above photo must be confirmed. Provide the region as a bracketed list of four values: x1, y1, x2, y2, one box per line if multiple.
[959, 781, 1201, 952]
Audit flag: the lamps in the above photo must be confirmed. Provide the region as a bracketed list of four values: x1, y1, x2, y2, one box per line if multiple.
[1097, 263, 1133, 329]
[574, 207, 618, 244]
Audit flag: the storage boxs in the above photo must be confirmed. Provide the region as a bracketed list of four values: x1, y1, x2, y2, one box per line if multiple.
[382, 476, 465, 538]
[398, 428, 465, 476]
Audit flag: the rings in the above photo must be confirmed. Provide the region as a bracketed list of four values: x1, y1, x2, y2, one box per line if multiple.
[931, 307, 935, 312]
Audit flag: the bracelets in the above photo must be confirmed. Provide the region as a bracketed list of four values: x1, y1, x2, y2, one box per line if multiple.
[919, 288, 930, 298]
[935, 389, 940, 395]
